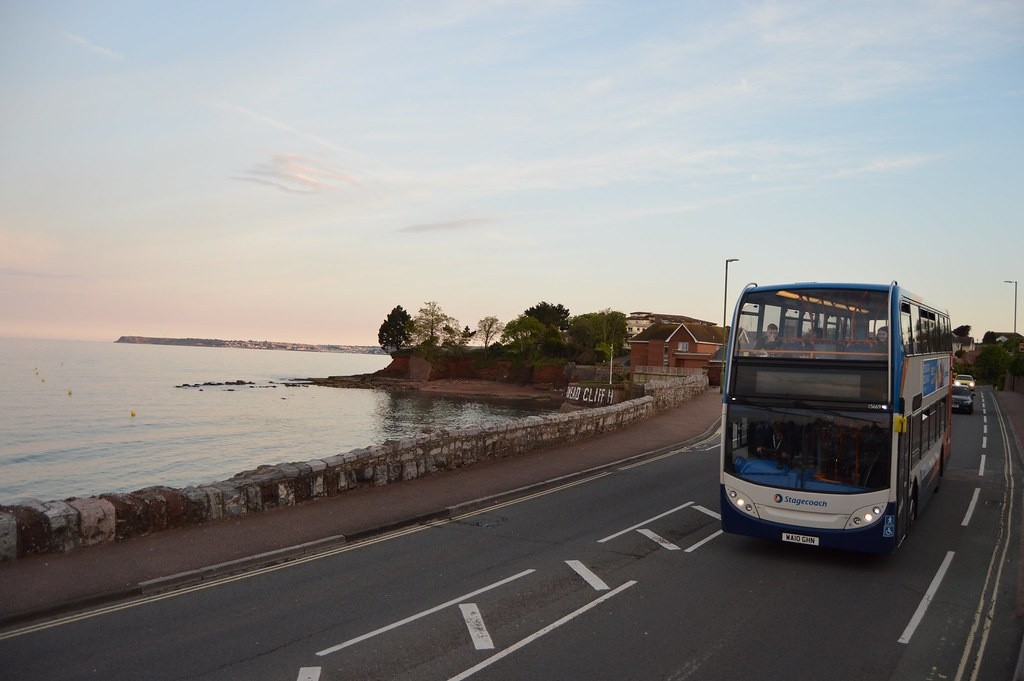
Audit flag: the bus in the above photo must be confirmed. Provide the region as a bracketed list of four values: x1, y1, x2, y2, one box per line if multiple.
[718, 281, 955, 556]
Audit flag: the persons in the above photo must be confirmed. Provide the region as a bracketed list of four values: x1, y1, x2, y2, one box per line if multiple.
[757, 419, 792, 459]
[754, 324, 784, 350]
[814, 328, 823, 339]
[876, 326, 888, 353]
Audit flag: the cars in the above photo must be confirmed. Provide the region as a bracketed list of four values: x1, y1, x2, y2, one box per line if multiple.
[951, 385, 976, 414]
[953, 374, 977, 391]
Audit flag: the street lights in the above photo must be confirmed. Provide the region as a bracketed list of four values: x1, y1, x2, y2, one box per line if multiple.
[720, 258, 741, 395]
[1003, 280, 1017, 332]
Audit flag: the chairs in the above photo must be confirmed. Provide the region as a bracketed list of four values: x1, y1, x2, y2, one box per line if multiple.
[753, 338, 934, 357]
